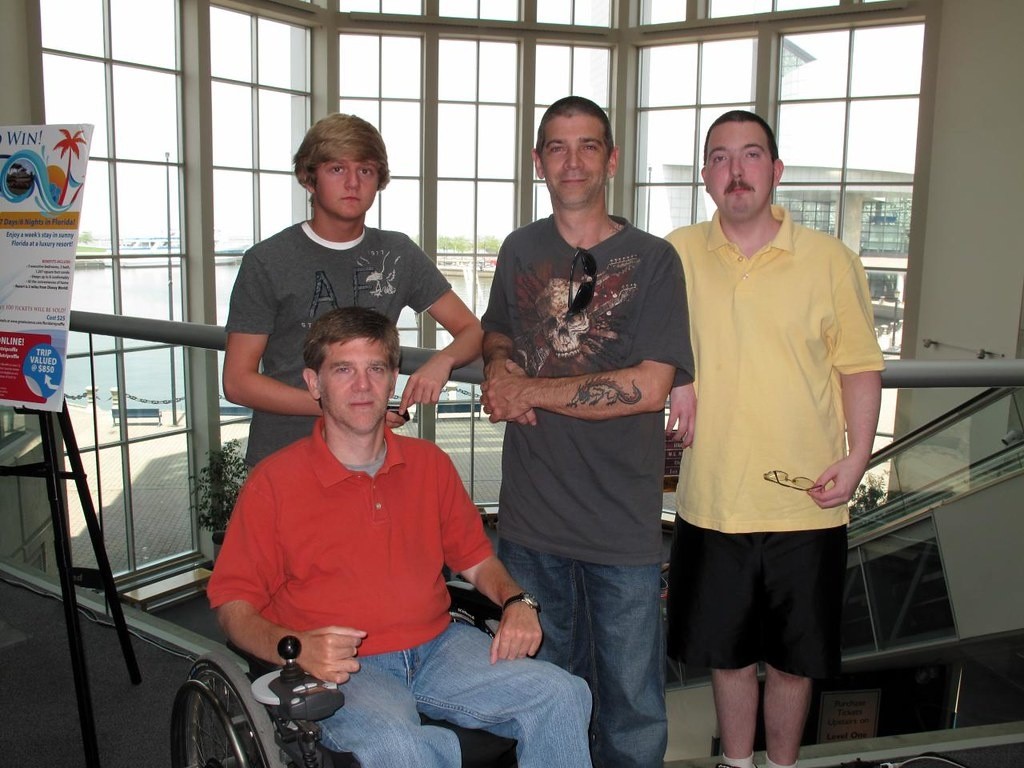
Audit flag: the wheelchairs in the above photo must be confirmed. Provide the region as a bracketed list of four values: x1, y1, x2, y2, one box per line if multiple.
[171, 580, 516, 768]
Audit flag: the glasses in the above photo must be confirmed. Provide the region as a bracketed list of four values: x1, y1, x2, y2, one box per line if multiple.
[763, 471, 822, 492]
[567, 247, 597, 316]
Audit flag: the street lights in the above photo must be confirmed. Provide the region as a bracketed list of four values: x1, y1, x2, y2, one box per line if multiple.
[892, 289, 900, 347]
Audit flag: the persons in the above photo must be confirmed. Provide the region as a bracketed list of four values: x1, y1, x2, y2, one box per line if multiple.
[221, 113, 485, 479]
[207, 306, 595, 768]
[660, 108, 883, 768]
[481, 96, 697, 768]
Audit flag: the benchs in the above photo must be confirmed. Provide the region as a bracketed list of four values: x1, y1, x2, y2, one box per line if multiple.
[220, 405, 252, 416]
[435, 402, 482, 423]
[111, 407, 164, 427]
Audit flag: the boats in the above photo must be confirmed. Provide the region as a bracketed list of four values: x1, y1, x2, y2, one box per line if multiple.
[96, 232, 253, 267]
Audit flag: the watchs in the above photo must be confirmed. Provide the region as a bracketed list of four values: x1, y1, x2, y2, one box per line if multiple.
[503, 590, 542, 614]
